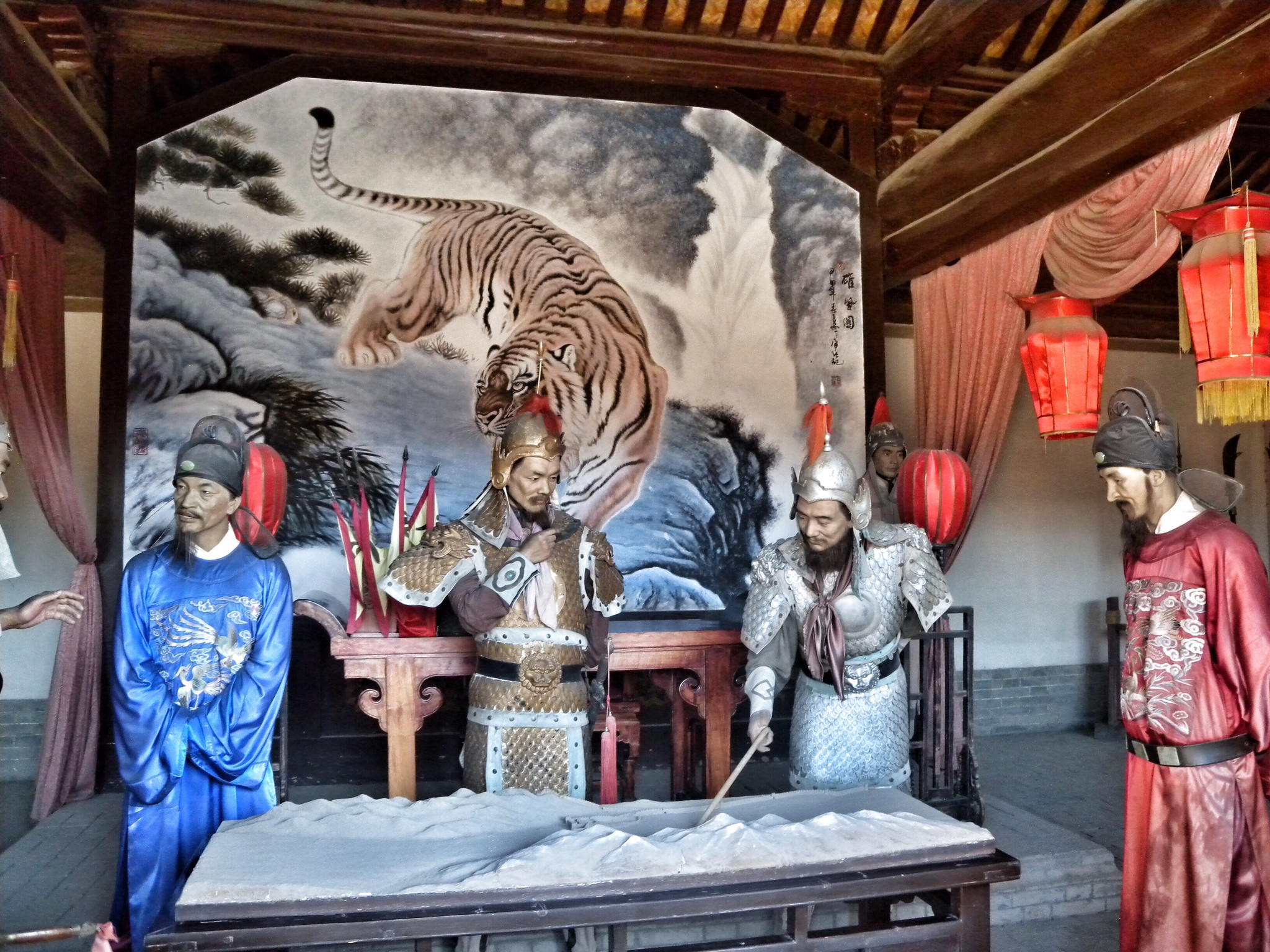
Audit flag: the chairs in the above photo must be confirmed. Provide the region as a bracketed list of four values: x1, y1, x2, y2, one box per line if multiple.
[591, 670, 640, 801]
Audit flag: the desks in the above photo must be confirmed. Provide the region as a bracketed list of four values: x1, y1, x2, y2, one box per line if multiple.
[144, 789, 1022, 952]
[291, 599, 748, 797]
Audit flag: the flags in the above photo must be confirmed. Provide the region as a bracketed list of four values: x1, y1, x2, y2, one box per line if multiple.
[334, 463, 440, 638]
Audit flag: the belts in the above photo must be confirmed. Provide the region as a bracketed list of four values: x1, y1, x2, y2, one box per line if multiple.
[472, 652, 585, 694]
[799, 649, 901, 692]
[1125, 730, 1260, 768]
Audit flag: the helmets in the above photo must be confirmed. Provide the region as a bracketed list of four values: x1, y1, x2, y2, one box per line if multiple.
[866, 390, 908, 462]
[489, 339, 566, 488]
[789, 380, 872, 530]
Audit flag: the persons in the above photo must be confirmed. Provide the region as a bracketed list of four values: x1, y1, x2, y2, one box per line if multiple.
[0, 422, 85, 714]
[861, 424, 905, 525]
[741, 452, 952, 799]
[115, 415, 293, 952]
[1093, 387, 1270, 952]
[385, 394, 626, 803]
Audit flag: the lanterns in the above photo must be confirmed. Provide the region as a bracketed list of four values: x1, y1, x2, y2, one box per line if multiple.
[235, 442, 286, 545]
[1155, 182, 1270, 427]
[1001, 286, 1116, 440]
[902, 445, 969, 547]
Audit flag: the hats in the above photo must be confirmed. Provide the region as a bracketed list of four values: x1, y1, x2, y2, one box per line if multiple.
[1088, 386, 1244, 512]
[129, 415, 285, 562]
